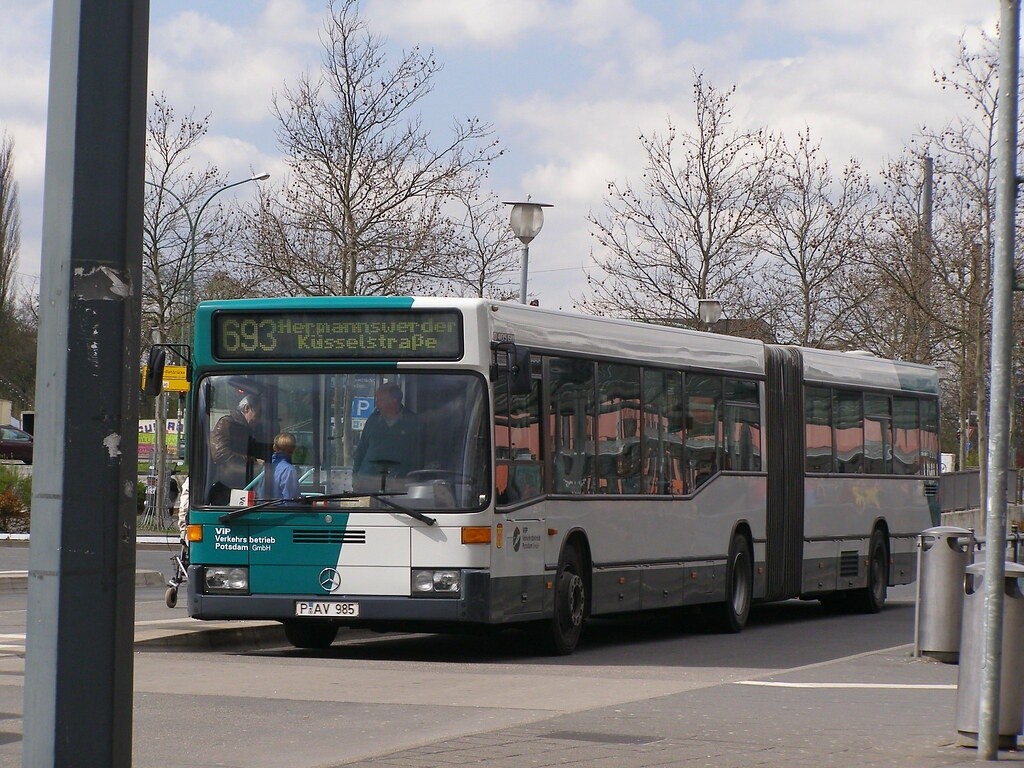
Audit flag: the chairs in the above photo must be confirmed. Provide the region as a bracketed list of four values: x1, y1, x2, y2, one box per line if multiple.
[500, 436, 940, 502]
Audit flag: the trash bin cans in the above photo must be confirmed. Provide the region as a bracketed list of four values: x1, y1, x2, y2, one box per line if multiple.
[912, 526, 975, 664]
[955, 561, 1024, 750]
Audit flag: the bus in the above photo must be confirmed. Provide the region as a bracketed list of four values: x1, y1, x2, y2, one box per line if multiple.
[142, 291, 944, 656]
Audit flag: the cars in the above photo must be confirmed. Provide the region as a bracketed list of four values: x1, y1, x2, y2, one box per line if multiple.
[0, 424, 33, 464]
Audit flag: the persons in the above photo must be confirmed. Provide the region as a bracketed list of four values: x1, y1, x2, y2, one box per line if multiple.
[179, 476, 188, 545]
[211, 392, 264, 494]
[351, 382, 414, 492]
[257, 432, 304, 505]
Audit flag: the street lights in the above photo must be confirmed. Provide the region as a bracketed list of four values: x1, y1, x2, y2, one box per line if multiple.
[501, 193, 555, 307]
[144, 172, 271, 372]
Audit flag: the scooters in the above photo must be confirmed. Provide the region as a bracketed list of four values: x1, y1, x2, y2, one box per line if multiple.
[143, 460, 185, 517]
